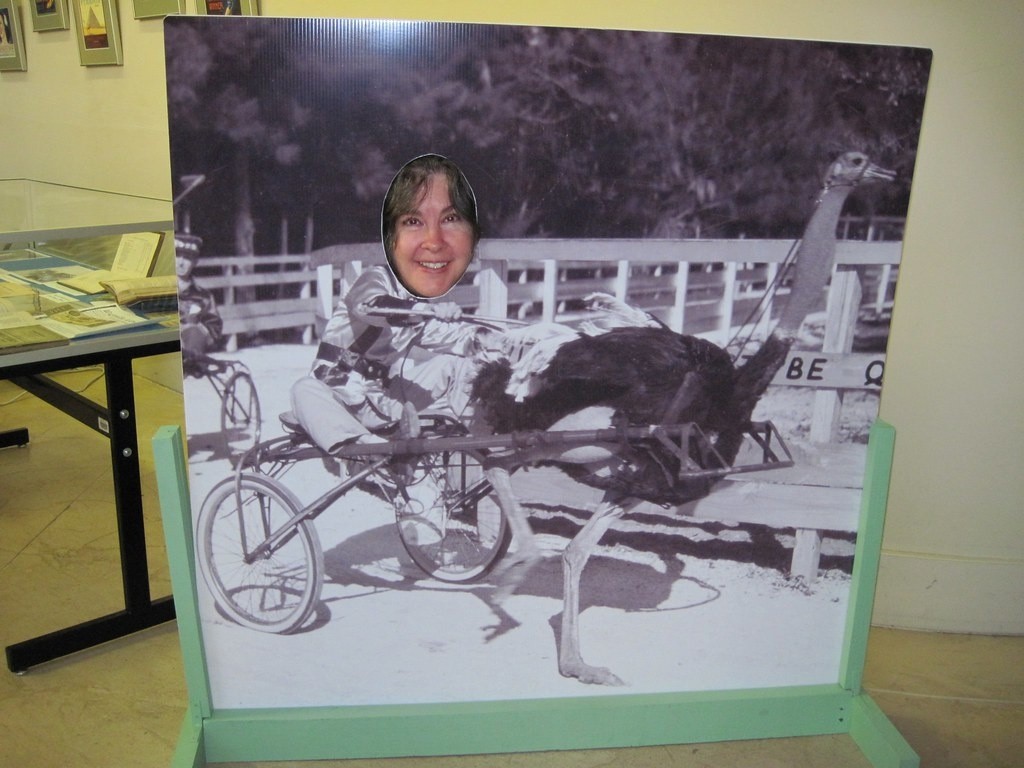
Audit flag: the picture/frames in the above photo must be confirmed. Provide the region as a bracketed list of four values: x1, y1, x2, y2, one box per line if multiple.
[0, 0, 27, 71]
[29, 0, 69, 32]
[72, 0, 124, 66]
[133, 0, 186, 20]
[194, 0, 258, 16]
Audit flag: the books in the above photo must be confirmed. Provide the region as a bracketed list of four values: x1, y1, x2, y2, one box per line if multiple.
[0, 230, 172, 318]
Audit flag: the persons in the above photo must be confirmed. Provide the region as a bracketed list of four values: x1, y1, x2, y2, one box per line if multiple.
[175, 231, 222, 374]
[293, 265, 490, 446]
[384, 155, 479, 297]
[0, 13, 12, 44]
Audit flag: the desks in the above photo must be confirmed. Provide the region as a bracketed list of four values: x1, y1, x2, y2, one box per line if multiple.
[0, 178, 184, 679]
